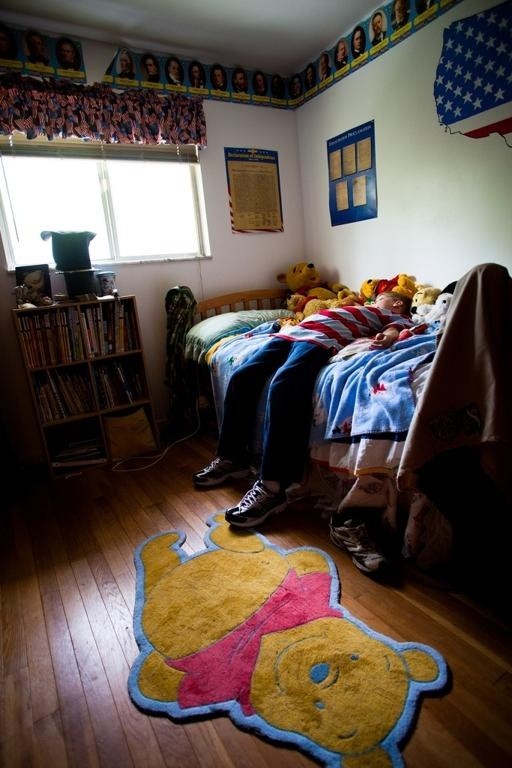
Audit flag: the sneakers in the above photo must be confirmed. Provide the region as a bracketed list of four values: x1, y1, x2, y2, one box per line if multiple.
[328, 512, 387, 575]
[192, 457, 251, 489]
[225, 480, 304, 528]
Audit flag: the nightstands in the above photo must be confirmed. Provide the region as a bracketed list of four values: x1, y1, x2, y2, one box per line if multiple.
[11, 295, 162, 482]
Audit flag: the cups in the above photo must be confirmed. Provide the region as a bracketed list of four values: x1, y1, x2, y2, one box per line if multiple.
[95, 271, 117, 295]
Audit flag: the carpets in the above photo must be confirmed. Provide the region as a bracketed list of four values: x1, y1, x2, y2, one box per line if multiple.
[128, 512, 449, 768]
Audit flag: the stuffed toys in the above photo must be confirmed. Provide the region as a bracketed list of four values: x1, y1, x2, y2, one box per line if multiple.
[275, 261, 453, 333]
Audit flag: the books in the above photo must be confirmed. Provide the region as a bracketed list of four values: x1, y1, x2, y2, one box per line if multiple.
[13, 295, 159, 473]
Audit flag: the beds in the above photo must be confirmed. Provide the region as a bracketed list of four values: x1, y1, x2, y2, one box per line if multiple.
[165, 262, 512, 572]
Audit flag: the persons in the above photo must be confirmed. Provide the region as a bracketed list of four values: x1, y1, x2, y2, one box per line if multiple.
[291, 37, 346, 100]
[391, 1, 409, 30]
[351, 26, 368, 57]
[2, 24, 81, 72]
[106, 49, 285, 100]
[195, 292, 407, 529]
[369, 12, 386, 46]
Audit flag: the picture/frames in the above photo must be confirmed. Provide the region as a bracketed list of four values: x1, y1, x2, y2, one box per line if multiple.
[15, 263, 53, 309]
